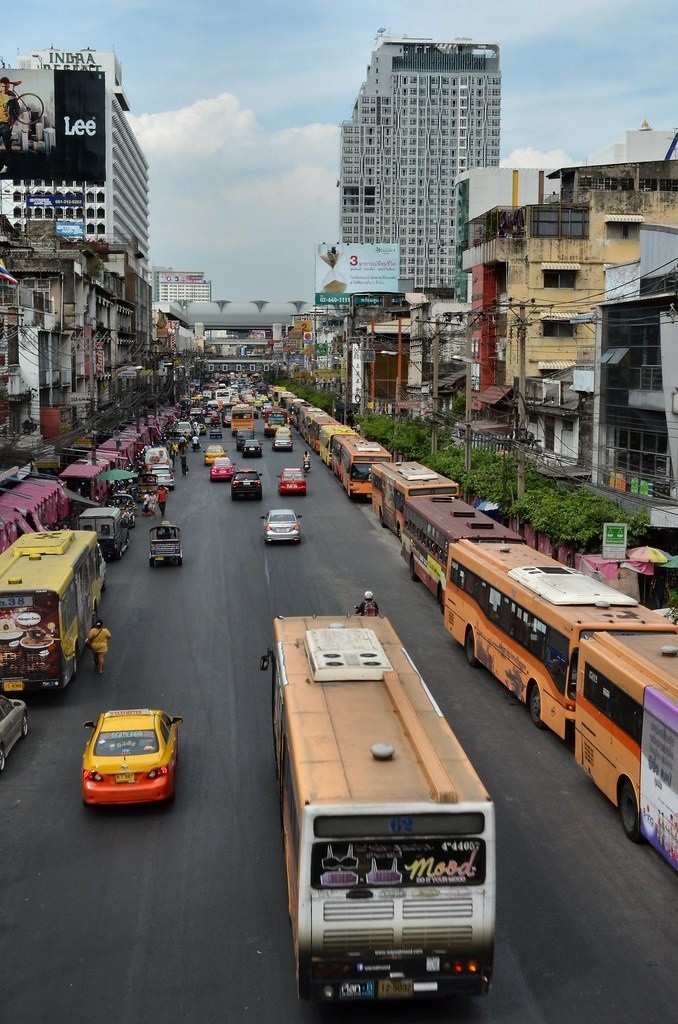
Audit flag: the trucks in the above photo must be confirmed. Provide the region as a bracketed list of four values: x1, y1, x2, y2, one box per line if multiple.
[77, 508, 130, 557]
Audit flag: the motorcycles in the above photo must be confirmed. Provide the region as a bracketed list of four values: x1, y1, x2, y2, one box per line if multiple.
[303, 458, 310, 471]
[148, 521, 183, 566]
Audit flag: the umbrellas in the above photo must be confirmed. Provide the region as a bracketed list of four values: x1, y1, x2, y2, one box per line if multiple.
[95, 469, 139, 481]
[627, 545, 678, 605]
[476, 499, 502, 511]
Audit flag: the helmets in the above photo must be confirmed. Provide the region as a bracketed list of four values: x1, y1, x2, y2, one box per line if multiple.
[364, 591, 373, 601]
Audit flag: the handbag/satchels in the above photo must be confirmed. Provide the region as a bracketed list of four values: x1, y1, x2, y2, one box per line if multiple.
[185, 466, 188, 472]
[86, 638, 92, 649]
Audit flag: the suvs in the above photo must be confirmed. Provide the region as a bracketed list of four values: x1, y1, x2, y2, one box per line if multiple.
[231, 468, 263, 500]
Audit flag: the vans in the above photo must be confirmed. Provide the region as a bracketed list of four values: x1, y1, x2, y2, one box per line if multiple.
[237, 429, 254, 450]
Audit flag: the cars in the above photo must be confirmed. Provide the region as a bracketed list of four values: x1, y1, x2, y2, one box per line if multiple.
[0, 695, 28, 771]
[137, 447, 174, 501]
[173, 390, 223, 440]
[203, 445, 237, 482]
[228, 377, 272, 418]
[83, 709, 183, 806]
[271, 427, 293, 451]
[261, 468, 307, 544]
[242, 439, 263, 457]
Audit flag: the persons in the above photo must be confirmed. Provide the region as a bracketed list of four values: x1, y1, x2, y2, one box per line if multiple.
[652, 572, 665, 609]
[0, 77, 20, 150]
[88, 619, 112, 674]
[302, 450, 312, 469]
[54, 523, 70, 530]
[169, 434, 200, 475]
[355, 591, 380, 618]
[105, 481, 168, 526]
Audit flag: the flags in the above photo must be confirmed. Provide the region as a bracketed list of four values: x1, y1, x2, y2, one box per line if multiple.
[0, 264, 19, 285]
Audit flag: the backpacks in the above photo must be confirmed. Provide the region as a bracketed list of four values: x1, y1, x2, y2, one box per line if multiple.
[364, 601, 377, 616]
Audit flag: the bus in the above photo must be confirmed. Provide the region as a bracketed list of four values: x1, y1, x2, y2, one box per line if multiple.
[217, 391, 253, 436]
[274, 387, 392, 500]
[370, 460, 678, 871]
[260, 614, 497, 998]
[265, 407, 287, 437]
[0, 531, 106, 693]
